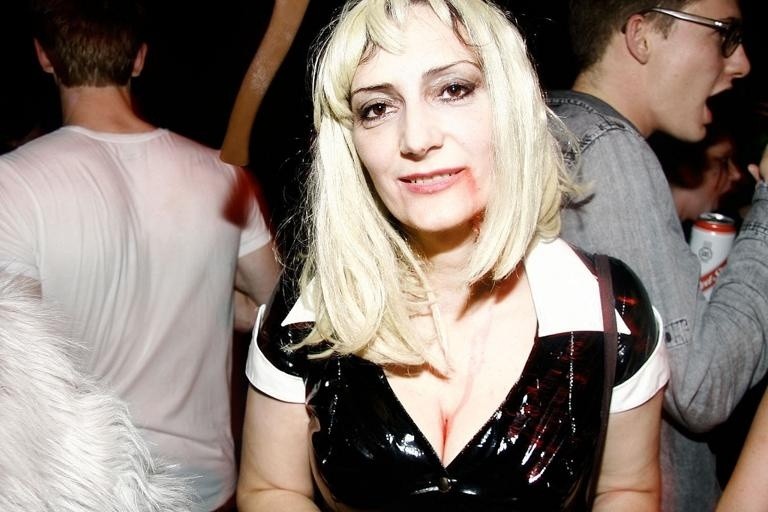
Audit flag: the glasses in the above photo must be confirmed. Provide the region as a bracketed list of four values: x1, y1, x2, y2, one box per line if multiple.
[621, 7, 744, 59]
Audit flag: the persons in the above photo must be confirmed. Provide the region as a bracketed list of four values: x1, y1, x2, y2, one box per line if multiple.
[234, 1, 675, 512]
[0, 0, 284, 512]
[660, 137, 742, 224]
[542, 2, 767, 510]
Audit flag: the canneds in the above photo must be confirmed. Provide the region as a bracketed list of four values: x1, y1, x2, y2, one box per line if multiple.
[689, 212, 738, 303]
